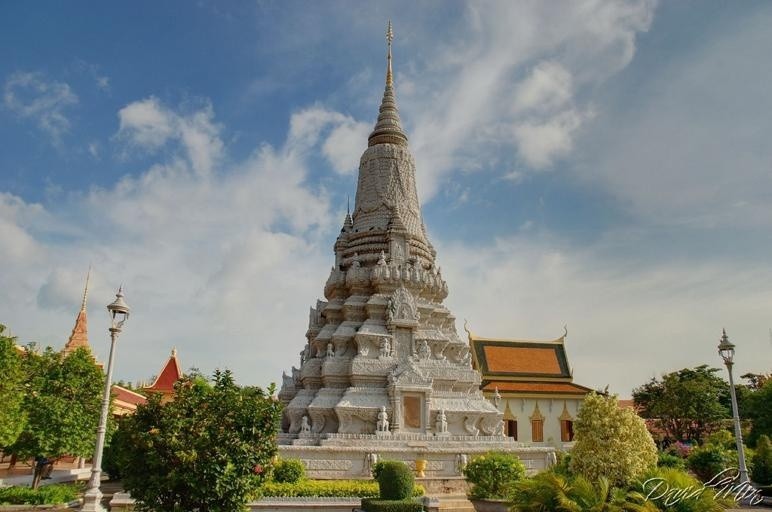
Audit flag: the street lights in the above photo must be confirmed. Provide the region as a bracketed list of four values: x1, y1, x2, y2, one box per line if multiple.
[718, 327, 759, 505]
[78, 284, 131, 511]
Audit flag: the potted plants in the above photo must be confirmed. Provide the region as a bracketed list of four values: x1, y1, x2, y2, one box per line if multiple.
[462, 446, 529, 512]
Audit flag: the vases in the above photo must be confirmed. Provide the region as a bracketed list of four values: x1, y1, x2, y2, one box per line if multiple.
[416, 459, 428, 478]
